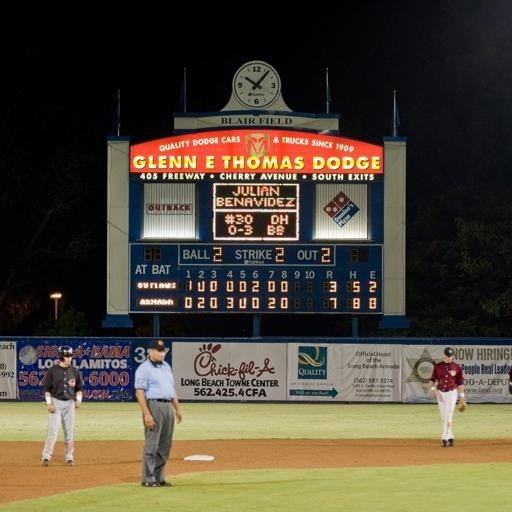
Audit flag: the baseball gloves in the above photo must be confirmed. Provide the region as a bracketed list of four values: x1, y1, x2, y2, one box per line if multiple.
[458, 400, 465, 412]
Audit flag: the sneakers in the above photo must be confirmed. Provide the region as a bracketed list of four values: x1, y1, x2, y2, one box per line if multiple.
[159, 481, 171, 486]
[67, 460, 73, 466]
[142, 482, 160, 486]
[43, 459, 49, 465]
[449, 439, 456, 446]
[441, 441, 447, 447]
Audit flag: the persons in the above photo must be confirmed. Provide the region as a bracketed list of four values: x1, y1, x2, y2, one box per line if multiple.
[429, 347, 465, 448]
[41, 346, 83, 467]
[133, 339, 183, 487]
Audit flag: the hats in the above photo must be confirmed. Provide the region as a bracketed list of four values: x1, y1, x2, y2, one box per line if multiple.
[445, 347, 453, 357]
[149, 340, 165, 351]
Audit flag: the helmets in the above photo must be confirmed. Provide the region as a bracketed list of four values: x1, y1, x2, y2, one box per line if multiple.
[58, 346, 74, 361]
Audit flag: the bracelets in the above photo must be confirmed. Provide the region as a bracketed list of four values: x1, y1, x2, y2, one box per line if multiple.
[431, 387, 436, 392]
[459, 393, 465, 397]
[45, 396, 51, 405]
[76, 395, 82, 402]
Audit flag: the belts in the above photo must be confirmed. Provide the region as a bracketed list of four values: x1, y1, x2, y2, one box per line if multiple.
[152, 398, 170, 402]
